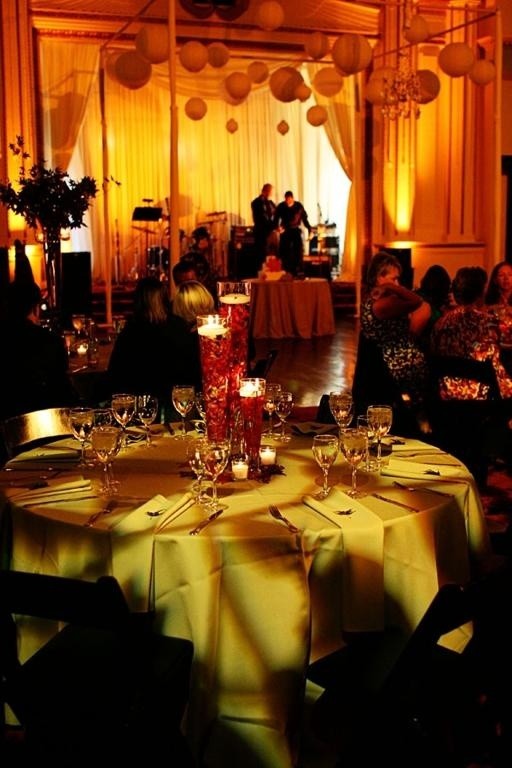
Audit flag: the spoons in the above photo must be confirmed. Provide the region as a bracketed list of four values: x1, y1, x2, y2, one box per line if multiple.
[147, 508, 168, 517]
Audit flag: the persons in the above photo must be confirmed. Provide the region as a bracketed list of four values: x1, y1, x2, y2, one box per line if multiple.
[225, 181, 312, 285]
[0, 226, 223, 414]
[362, 251, 512, 401]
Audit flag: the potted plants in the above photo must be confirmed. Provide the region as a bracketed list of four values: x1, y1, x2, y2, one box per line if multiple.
[1, 133, 121, 312]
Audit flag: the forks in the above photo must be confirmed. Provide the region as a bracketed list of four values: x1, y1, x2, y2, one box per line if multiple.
[394, 452, 448, 457]
[6, 472, 60, 482]
[41, 445, 90, 455]
[84, 499, 118, 528]
[268, 504, 301, 534]
[394, 481, 453, 498]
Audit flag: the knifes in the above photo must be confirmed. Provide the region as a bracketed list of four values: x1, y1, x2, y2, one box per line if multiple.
[370, 491, 420, 513]
[22, 496, 98, 508]
[189, 509, 224, 537]
[4, 466, 64, 472]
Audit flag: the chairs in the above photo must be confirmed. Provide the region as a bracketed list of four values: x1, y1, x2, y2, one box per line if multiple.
[0, 563, 199, 766]
[305, 573, 512, 767]
[0, 569, 197, 692]
[4, 405, 94, 457]
[343, 580, 511, 689]
[424, 353, 512, 465]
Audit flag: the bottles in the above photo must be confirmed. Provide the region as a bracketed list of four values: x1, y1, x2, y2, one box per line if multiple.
[86, 321, 100, 367]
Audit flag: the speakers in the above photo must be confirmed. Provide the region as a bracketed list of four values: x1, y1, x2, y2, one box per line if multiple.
[135, 276, 162, 291]
[380, 247, 411, 288]
[304, 255, 334, 277]
[58, 253, 93, 330]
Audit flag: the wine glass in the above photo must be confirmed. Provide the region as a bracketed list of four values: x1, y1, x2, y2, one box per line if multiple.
[328, 391, 392, 497]
[170, 377, 295, 512]
[312, 434, 339, 498]
[69, 394, 158, 494]
[63, 313, 88, 358]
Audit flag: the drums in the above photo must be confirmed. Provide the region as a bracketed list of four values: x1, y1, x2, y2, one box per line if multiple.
[145, 245, 170, 272]
